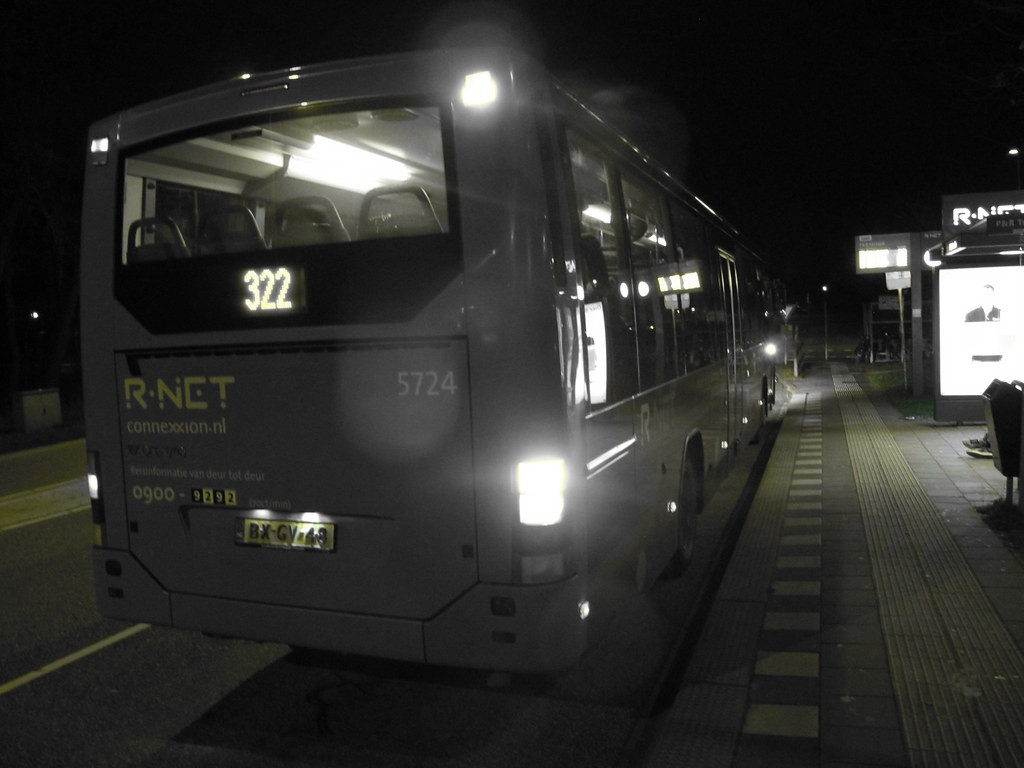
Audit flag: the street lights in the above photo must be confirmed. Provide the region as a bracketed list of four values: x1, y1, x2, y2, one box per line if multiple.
[822, 286, 827, 360]
[1008, 148, 1021, 190]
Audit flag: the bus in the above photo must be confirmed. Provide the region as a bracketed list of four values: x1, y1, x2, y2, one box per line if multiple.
[81, 45, 777, 675]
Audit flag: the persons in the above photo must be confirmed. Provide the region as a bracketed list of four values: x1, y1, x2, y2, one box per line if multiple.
[966, 285, 1002, 361]
[855, 331, 892, 361]
[963, 433, 992, 458]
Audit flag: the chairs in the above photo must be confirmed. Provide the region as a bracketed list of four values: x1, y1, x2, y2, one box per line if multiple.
[358, 184, 443, 239]
[192, 204, 266, 257]
[272, 195, 350, 247]
[126, 216, 190, 265]
